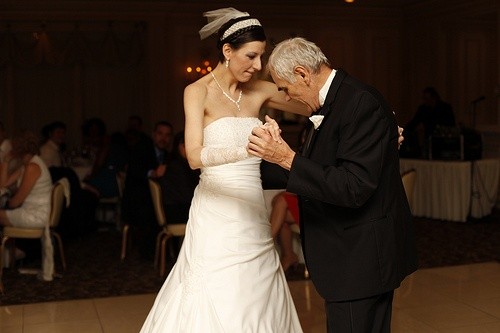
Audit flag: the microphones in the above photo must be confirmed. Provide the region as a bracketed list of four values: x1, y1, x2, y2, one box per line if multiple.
[470, 96, 485, 105]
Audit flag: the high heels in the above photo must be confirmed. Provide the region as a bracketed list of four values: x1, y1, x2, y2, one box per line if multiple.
[280, 253, 298, 273]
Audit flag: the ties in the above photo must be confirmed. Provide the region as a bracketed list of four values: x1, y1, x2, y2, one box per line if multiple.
[302, 109, 323, 157]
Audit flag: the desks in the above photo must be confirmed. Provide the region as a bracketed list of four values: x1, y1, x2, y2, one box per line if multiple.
[399, 157, 500, 223]
[72, 165, 93, 184]
[263, 189, 309, 279]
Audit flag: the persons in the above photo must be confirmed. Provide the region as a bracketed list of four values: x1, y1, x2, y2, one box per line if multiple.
[137, 8, 405, 333]
[246, 37, 414, 333]
[407, 87, 455, 142]
[0, 115, 301, 277]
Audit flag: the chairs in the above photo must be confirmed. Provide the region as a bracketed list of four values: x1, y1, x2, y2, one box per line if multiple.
[115, 173, 129, 261]
[96, 197, 117, 234]
[0, 177, 69, 276]
[400, 168, 417, 212]
[148, 179, 188, 276]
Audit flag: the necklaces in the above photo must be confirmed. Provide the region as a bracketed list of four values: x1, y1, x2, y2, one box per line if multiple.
[211, 71, 242, 111]
